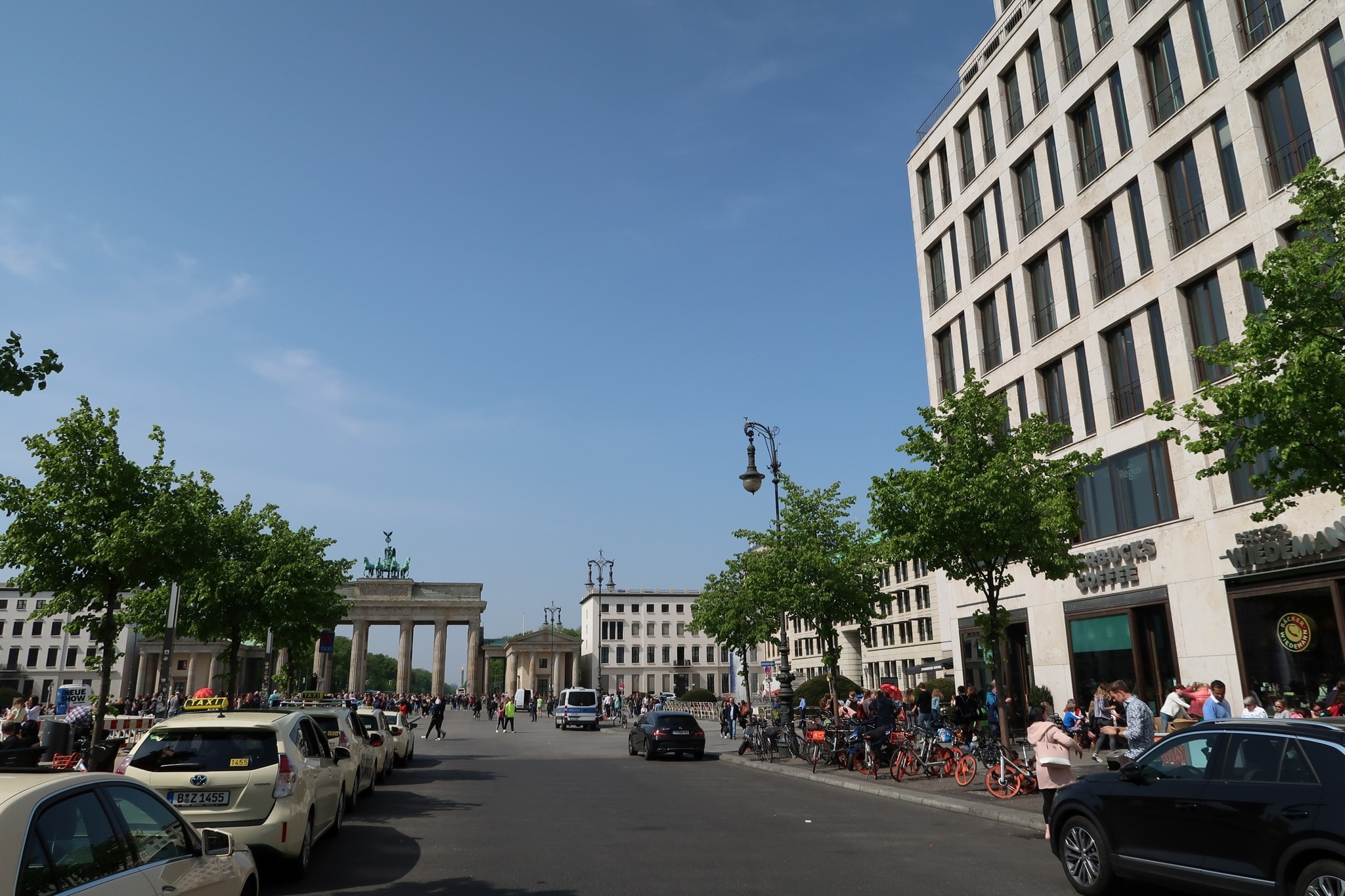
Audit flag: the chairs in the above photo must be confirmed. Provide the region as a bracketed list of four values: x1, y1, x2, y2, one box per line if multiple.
[1154, 716, 1198, 738]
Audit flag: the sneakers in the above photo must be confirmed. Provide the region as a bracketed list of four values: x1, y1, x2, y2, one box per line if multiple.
[503, 729, 507, 733]
[1092, 752, 1103, 762]
[724, 736, 728, 739]
[730, 737, 732, 739]
[733, 736, 737, 740]
[919, 738, 924, 743]
[442, 732, 446, 739]
[512, 730, 517, 733]
[421, 735, 427, 740]
[496, 730, 499, 732]
[435, 738, 441, 741]
[721, 734, 723, 737]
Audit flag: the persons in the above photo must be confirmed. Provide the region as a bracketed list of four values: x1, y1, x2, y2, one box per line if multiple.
[718, 697, 748, 739]
[797, 695, 806, 728]
[0, 690, 555, 768]
[388, 547, 392, 558]
[1027, 681, 1345, 841]
[819, 679, 1012, 748]
[603, 692, 667, 719]
[772, 693, 781, 727]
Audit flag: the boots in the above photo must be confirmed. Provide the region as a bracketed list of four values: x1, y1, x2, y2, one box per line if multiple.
[1045, 824, 1050, 840]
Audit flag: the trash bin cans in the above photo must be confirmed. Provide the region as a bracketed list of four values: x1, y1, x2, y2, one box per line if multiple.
[1106, 748, 1130, 774]
[41, 718, 71, 762]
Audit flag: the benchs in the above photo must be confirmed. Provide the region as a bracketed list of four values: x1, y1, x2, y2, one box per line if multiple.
[72, 737, 130, 772]
[0, 745, 51, 768]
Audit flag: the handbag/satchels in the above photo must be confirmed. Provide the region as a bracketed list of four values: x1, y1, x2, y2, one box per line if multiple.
[1036, 730, 1071, 770]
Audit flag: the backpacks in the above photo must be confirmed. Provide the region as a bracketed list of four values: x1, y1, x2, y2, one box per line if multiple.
[975, 704, 988, 720]
[958, 702, 975, 718]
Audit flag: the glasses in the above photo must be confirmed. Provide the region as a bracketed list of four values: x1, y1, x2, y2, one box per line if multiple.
[1275, 705, 1282, 708]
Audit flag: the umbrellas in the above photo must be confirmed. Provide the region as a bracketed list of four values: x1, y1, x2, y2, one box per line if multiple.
[880, 683, 902, 701]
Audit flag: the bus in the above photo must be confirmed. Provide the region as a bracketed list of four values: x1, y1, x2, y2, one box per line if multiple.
[455, 688, 466, 696]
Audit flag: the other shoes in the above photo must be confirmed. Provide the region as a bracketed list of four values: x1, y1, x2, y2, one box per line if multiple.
[489, 718, 490, 720]
[473, 715, 476, 717]
[796, 726, 801, 728]
[529, 715, 555, 722]
[629, 715, 639, 719]
[420, 714, 431, 718]
[452, 708, 472, 712]
[493, 713, 494, 716]
[606, 716, 618, 720]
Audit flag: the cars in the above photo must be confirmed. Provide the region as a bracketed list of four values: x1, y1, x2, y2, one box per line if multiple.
[1047, 717, 1345, 895]
[0, 767, 260, 896]
[115, 693, 351, 880]
[629, 711, 706, 761]
[260, 699, 385, 816]
[382, 710, 417, 768]
[354, 706, 400, 785]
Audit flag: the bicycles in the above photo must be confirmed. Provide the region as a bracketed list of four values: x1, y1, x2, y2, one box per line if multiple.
[613, 708, 628, 729]
[742, 707, 1040, 799]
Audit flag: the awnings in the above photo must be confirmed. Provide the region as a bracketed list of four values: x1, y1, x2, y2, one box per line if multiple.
[906, 658, 953, 674]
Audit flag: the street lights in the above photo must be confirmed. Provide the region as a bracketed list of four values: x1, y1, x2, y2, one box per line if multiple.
[541, 599, 563, 712]
[739, 416, 799, 759]
[584, 547, 617, 721]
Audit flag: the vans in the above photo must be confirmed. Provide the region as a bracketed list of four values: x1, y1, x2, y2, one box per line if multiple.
[512, 688, 533, 713]
[552, 687, 599, 730]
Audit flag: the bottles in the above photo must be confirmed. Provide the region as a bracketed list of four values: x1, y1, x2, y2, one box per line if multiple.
[1268, 684, 1273, 695]
[1272, 683, 1277, 695]
[6, 708, 10, 713]
[1276, 684, 1280, 695]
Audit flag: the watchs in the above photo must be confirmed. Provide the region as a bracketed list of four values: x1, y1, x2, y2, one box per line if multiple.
[1116, 729, 1120, 735]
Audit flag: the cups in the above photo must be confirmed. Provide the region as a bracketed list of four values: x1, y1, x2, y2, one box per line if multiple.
[1260, 682, 1268, 691]
